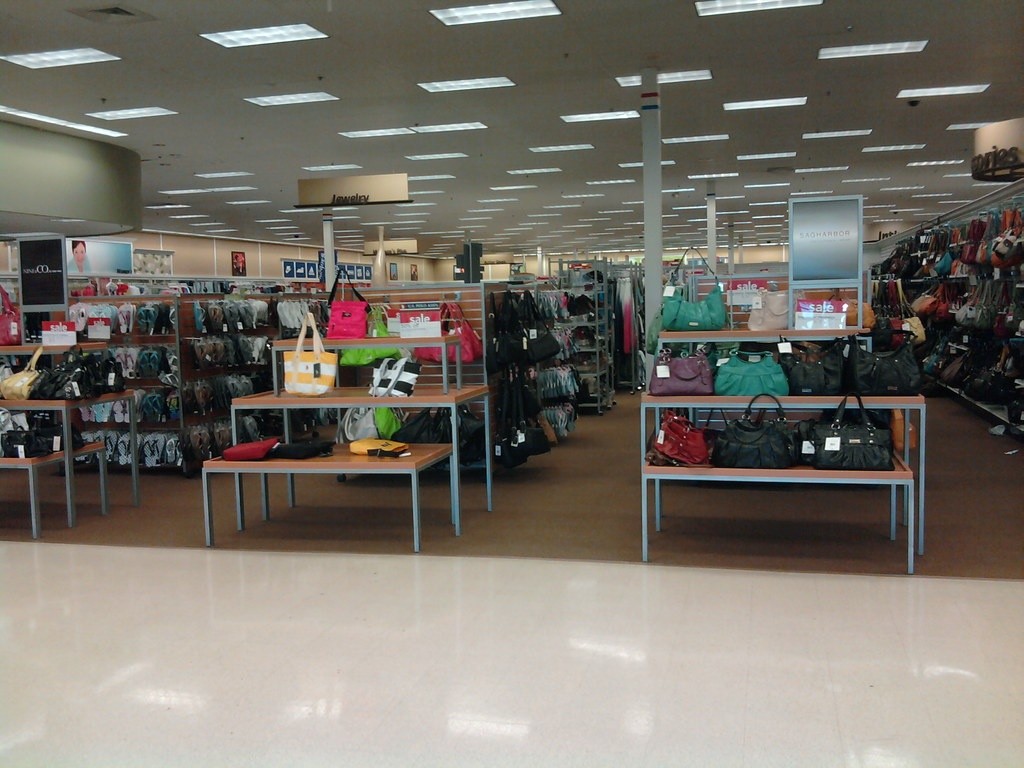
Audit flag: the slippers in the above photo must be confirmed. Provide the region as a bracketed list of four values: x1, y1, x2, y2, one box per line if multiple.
[69, 301, 181, 468]
[182, 297, 272, 461]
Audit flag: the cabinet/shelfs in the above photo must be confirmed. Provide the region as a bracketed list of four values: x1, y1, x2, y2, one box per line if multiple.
[0, 388, 141, 526]
[2, 255, 642, 481]
[868, 192, 1024, 436]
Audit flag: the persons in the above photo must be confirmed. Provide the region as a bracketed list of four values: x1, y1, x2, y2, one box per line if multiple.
[67, 240, 93, 273]
[233, 254, 246, 274]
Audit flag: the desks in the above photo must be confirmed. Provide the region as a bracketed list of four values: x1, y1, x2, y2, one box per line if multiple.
[201, 444, 455, 553]
[231, 383, 492, 535]
[0, 341, 108, 362]
[0, 441, 109, 539]
[639, 392, 926, 556]
[642, 461, 915, 576]
[267, 334, 463, 396]
[658, 329, 873, 353]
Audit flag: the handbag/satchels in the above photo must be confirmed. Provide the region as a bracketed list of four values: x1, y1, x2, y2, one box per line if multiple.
[643, 247, 926, 470]
[326, 267, 371, 339]
[284, 313, 338, 397]
[340, 306, 414, 367]
[485, 287, 557, 468]
[368, 357, 422, 397]
[0, 285, 89, 458]
[222, 405, 484, 470]
[872, 206, 1024, 426]
[414, 302, 483, 364]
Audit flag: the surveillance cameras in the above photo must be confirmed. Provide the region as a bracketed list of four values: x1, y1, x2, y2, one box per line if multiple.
[767, 241, 771, 243]
[908, 100, 920, 107]
[894, 212, 898, 214]
